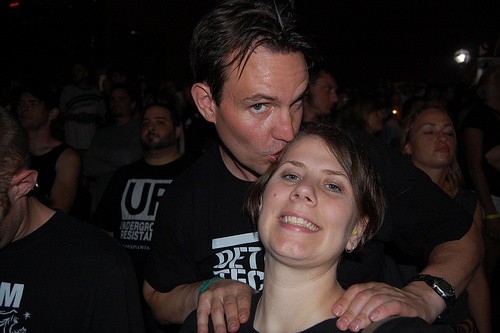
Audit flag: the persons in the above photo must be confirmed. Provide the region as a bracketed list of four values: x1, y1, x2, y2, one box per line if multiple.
[141, 0, 486, 333]
[0, 40, 500, 333]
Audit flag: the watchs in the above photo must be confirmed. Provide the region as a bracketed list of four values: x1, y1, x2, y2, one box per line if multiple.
[408, 274, 457, 309]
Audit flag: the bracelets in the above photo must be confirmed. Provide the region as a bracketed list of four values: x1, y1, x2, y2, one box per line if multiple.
[198, 275, 222, 301]
